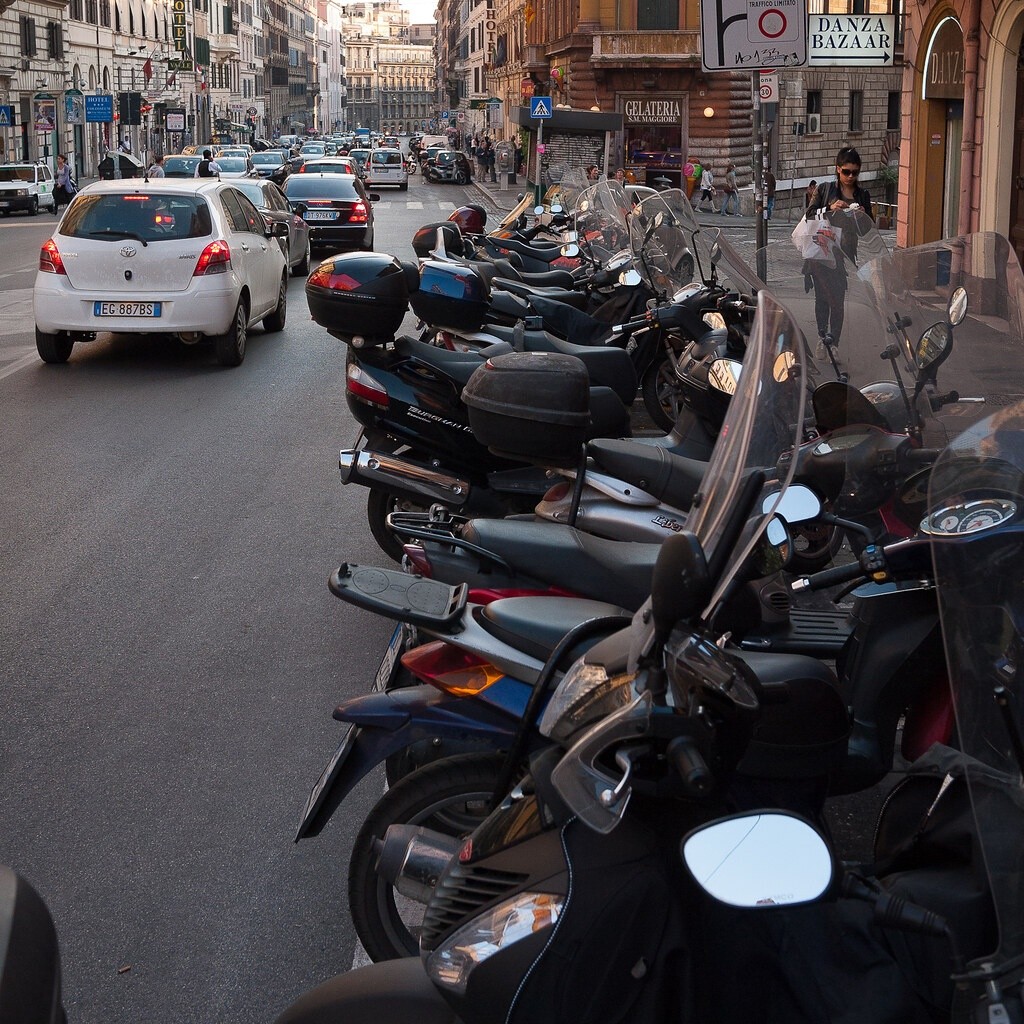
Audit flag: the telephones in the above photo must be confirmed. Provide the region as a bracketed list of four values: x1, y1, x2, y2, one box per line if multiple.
[500, 152, 508, 163]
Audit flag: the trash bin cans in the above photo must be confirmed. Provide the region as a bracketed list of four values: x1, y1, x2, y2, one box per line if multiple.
[97, 151, 145, 180]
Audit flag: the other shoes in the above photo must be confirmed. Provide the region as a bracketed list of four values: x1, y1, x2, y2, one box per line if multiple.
[734, 213, 743, 217]
[722, 214, 730, 216]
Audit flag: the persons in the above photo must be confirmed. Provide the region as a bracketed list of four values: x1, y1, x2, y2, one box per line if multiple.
[124, 136, 130, 149]
[36, 105, 53, 124]
[466, 136, 496, 183]
[53, 154, 74, 216]
[194, 150, 222, 179]
[616, 167, 625, 183]
[148, 155, 164, 178]
[694, 163, 720, 214]
[806, 146, 873, 365]
[586, 164, 600, 180]
[765, 172, 776, 220]
[721, 164, 742, 217]
[807, 180, 817, 202]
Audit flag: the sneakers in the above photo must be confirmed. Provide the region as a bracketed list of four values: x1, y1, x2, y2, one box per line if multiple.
[829, 345, 841, 364]
[816, 336, 827, 360]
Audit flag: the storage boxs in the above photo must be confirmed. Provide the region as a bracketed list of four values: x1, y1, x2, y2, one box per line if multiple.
[304, 251, 407, 342]
[459, 350, 590, 466]
[446, 205, 487, 235]
[412, 221, 464, 260]
[410, 260, 493, 332]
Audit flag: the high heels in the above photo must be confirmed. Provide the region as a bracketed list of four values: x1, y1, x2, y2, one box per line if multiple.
[51, 209, 58, 215]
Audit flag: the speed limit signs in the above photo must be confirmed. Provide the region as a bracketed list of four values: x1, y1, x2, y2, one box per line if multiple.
[760, 84, 773, 99]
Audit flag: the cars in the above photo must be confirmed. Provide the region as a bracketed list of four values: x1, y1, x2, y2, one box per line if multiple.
[277, 171, 381, 254]
[164, 126, 471, 185]
[214, 177, 315, 279]
[33, 177, 291, 370]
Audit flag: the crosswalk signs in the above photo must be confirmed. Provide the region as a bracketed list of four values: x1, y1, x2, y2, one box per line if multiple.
[0, 106, 11, 126]
[529, 96, 554, 119]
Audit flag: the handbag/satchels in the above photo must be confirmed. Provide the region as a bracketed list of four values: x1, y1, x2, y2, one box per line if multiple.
[792, 182, 832, 252]
[801, 214, 843, 270]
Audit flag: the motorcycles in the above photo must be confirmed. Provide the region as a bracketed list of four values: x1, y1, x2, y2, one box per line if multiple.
[262, 155, 1024, 1024]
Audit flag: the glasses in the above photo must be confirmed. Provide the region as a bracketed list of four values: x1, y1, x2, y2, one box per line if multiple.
[840, 167, 859, 176]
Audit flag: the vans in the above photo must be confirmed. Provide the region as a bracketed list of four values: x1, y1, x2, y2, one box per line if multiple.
[0, 160, 57, 215]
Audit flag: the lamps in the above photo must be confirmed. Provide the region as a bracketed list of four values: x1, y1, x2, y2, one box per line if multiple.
[10, 56, 30, 70]
[64, 77, 87, 86]
[36, 77, 49, 88]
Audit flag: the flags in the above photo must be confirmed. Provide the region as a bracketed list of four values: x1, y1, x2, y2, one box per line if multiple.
[144, 59, 152, 83]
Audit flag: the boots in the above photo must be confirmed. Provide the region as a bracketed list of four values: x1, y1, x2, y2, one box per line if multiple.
[709, 200, 720, 214]
[695, 199, 703, 213]
